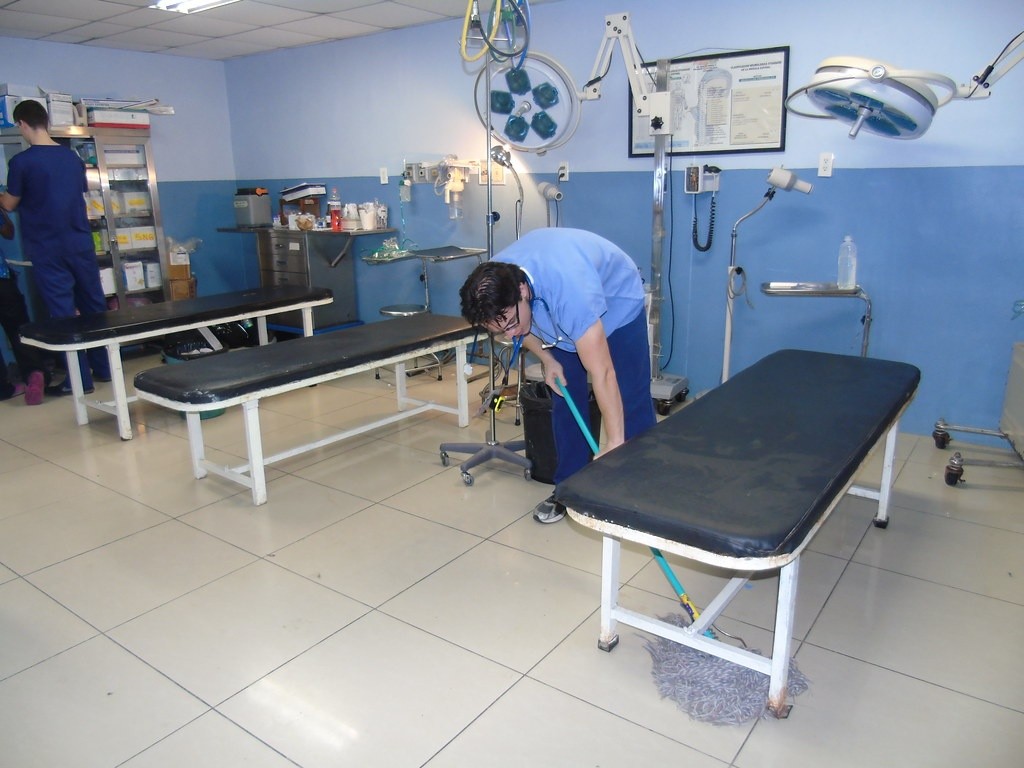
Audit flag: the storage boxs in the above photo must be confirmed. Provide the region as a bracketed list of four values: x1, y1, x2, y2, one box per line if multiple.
[0, 80, 165, 295]
[233, 182, 329, 230]
[169, 275, 197, 301]
[167, 250, 191, 281]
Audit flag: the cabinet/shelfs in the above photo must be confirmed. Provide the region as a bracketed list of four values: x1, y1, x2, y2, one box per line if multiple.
[0, 125, 169, 311]
[217, 227, 398, 332]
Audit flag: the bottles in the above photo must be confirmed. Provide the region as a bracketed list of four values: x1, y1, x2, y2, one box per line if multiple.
[399, 179, 411, 202]
[444, 180, 464, 218]
[837, 235, 856, 290]
[330, 188, 343, 232]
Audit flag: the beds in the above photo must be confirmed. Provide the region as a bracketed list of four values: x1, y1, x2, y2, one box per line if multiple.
[130, 315, 491, 506]
[547, 347, 925, 717]
[19, 285, 334, 439]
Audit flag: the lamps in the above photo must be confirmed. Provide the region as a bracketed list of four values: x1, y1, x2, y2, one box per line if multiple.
[537, 181, 564, 228]
[722, 165, 812, 384]
[490, 145, 525, 243]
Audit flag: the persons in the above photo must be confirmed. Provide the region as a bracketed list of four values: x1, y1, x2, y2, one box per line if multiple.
[0, 210, 54, 405]
[0, 100, 111, 396]
[459, 228, 657, 525]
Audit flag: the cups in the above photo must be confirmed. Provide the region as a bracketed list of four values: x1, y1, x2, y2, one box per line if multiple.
[359, 210, 376, 231]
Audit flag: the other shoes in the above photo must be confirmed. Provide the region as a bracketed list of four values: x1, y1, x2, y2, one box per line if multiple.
[9, 384, 26, 398]
[25, 371, 44, 405]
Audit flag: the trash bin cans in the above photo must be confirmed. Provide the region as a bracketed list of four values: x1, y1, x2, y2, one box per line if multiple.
[519, 381, 601, 485]
[160, 339, 230, 420]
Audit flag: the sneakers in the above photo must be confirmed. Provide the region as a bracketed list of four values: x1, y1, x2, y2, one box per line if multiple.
[92, 374, 112, 382]
[46, 381, 93, 395]
[533, 495, 567, 524]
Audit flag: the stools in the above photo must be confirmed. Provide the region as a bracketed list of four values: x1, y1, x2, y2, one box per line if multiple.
[480, 331, 541, 426]
[373, 304, 444, 382]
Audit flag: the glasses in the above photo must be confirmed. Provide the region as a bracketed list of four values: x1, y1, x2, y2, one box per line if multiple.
[486, 300, 519, 337]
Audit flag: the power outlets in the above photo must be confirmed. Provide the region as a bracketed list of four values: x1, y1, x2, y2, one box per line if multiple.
[558, 161, 570, 182]
[817, 151, 834, 177]
